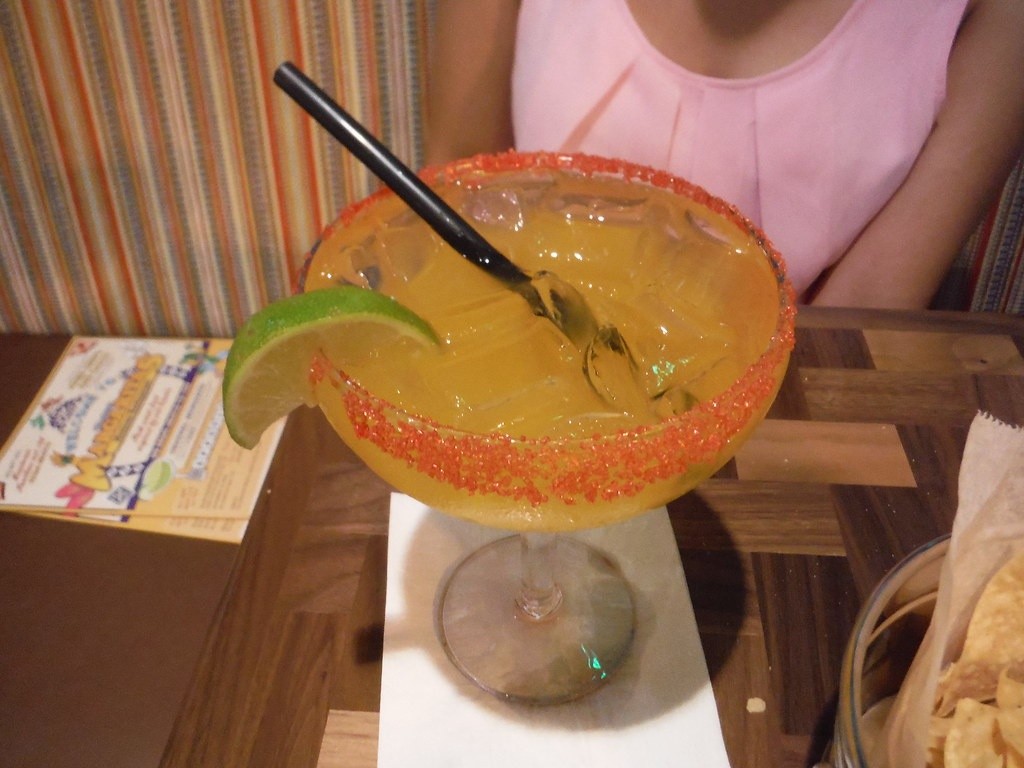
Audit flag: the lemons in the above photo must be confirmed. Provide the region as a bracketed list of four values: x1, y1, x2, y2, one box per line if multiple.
[219, 284, 438, 451]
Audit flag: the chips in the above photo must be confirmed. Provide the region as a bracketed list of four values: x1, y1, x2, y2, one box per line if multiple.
[923, 539, 1024, 768]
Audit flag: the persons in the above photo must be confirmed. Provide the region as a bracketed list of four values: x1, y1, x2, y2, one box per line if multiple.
[425, 0, 1022, 320]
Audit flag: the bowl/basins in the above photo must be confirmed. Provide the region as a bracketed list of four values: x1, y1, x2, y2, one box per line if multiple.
[828, 527, 981, 768]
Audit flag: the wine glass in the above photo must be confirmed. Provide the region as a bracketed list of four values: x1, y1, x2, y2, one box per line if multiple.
[290, 143, 798, 712]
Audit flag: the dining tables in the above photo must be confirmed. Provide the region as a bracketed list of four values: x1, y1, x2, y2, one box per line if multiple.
[0, 307, 1024, 768]
